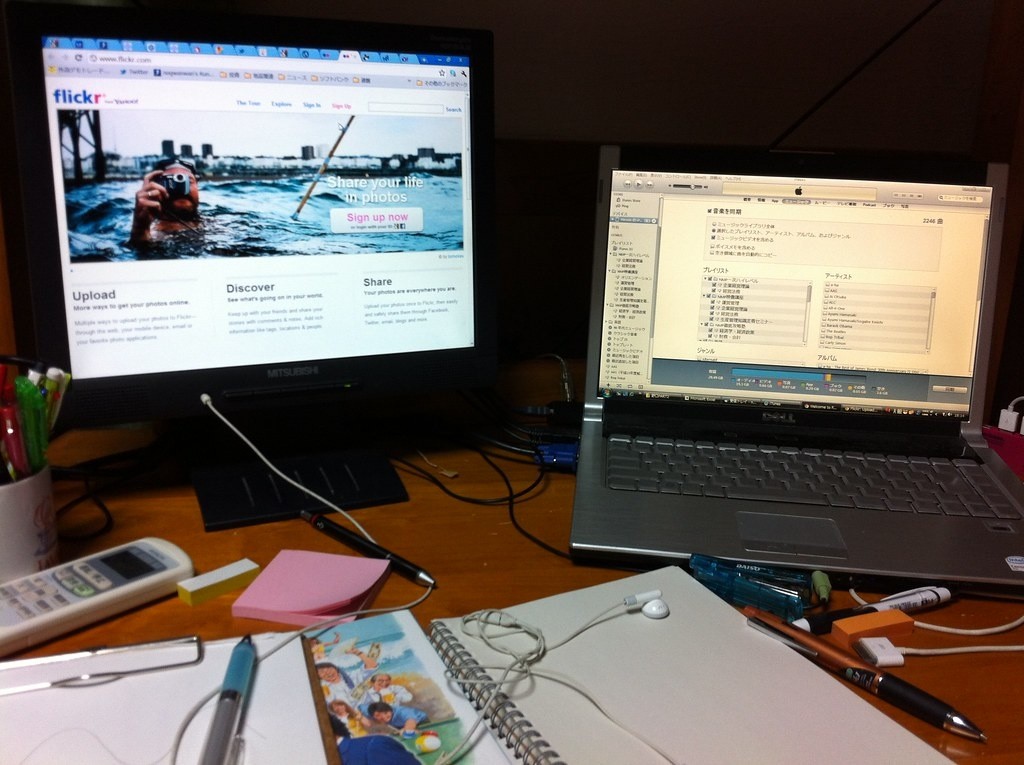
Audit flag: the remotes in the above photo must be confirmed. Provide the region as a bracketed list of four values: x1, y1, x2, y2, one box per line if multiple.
[0, 536, 194, 663]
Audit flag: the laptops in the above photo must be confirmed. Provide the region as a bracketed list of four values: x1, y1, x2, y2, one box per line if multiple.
[562, 142, 1023, 604]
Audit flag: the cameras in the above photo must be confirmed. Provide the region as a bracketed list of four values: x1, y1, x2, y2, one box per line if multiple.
[153, 173, 190, 202]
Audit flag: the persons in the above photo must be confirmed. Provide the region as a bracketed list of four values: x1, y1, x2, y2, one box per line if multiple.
[131, 158, 204, 244]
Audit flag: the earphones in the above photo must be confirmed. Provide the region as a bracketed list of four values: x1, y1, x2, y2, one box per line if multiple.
[624, 588, 670, 620]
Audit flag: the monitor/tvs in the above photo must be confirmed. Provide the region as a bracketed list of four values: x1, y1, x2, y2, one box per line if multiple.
[0, 3, 497, 445]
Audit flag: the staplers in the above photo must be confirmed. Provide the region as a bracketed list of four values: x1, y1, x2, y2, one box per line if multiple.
[686, 552, 814, 623]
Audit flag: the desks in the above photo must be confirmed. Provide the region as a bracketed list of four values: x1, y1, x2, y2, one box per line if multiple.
[1, 357, 1024, 765]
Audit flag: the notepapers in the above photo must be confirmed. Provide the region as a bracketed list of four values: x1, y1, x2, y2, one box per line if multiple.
[176, 556, 261, 607]
[231, 549, 392, 630]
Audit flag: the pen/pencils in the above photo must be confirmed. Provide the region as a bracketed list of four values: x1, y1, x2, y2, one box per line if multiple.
[790, 585, 958, 636]
[299, 508, 438, 591]
[197, 632, 258, 765]
[0, 362, 70, 488]
[741, 605, 989, 743]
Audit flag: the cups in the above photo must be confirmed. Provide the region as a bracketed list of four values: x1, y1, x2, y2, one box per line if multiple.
[1, 460, 55, 588]
